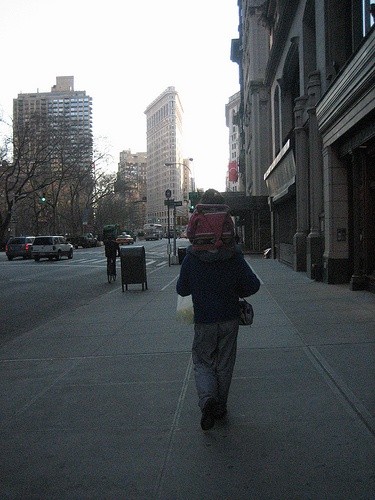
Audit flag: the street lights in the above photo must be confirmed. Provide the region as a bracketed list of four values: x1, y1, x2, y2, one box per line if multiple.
[164, 162, 195, 211]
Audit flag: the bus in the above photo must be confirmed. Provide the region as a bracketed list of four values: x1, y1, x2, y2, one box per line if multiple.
[143, 224, 163, 239]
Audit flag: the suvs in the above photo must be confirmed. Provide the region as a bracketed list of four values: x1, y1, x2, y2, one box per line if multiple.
[73, 232, 98, 249]
[5, 236, 36, 260]
[30, 236, 74, 261]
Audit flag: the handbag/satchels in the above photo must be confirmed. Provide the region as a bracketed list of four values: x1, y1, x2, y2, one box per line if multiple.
[237, 300, 253, 325]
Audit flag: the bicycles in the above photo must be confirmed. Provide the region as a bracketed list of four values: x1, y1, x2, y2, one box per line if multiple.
[106, 255, 121, 284]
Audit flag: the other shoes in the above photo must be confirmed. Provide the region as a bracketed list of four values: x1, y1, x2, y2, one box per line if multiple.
[200, 397, 217, 431]
[217, 407, 228, 419]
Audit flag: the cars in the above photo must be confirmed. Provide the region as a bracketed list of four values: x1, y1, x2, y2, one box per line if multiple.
[144, 232, 160, 241]
[115, 232, 134, 245]
[165, 229, 180, 238]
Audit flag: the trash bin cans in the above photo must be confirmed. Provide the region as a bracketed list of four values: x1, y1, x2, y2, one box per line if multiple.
[120, 246, 146, 284]
[177, 247, 186, 265]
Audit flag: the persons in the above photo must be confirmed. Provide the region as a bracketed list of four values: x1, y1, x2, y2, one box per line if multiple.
[176, 252, 261, 430]
[105, 236, 120, 276]
[234, 232, 240, 244]
[185, 188, 236, 260]
